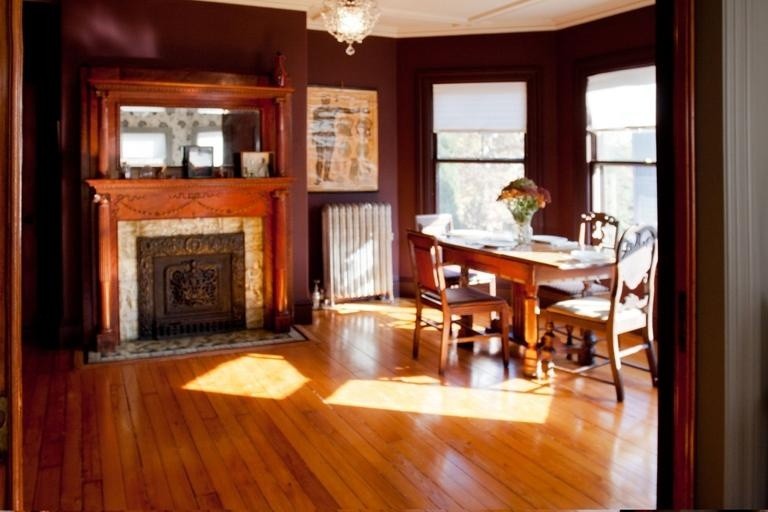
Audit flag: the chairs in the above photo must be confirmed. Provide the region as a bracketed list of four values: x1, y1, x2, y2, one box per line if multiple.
[541, 222, 658, 403]
[537, 211, 618, 364]
[405, 228, 510, 378]
[415, 214, 477, 336]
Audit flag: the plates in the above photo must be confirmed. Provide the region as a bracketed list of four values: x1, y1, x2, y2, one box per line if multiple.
[571, 250, 604, 263]
[533, 234, 568, 244]
[447, 228, 491, 238]
[477, 240, 511, 248]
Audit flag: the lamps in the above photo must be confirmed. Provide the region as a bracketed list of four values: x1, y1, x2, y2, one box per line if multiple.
[312, 1, 380, 55]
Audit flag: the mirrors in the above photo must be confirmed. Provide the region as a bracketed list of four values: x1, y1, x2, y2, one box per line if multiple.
[95, 90, 285, 177]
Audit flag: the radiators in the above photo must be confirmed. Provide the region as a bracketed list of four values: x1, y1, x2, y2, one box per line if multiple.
[321, 201, 395, 306]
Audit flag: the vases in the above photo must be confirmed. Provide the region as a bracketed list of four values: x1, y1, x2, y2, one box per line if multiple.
[517, 224, 532, 247]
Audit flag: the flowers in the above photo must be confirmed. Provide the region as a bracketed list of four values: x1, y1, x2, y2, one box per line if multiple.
[496, 178, 550, 224]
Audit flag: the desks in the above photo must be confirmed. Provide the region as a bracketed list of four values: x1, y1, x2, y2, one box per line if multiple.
[435, 229, 615, 378]
